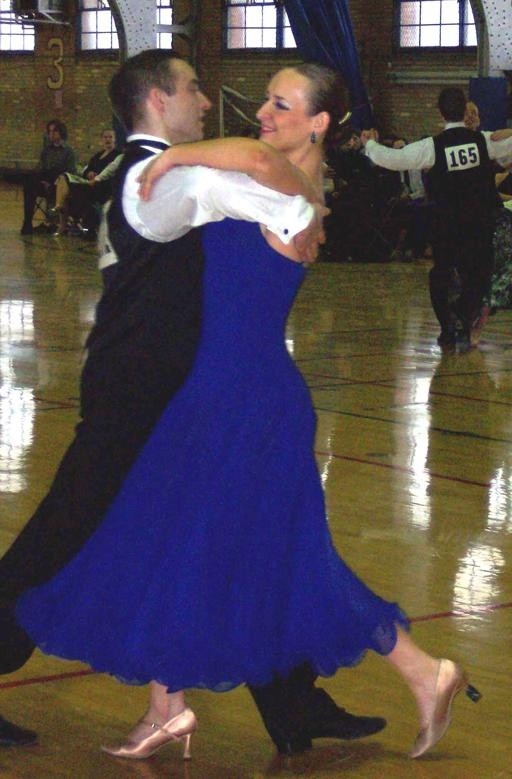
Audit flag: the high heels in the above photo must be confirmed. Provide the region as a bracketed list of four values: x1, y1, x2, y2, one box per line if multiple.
[103, 706, 199, 762]
[409, 659, 480, 758]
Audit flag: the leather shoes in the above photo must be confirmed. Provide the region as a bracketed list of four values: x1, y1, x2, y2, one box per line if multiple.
[0, 718, 36, 747]
[279, 706, 388, 755]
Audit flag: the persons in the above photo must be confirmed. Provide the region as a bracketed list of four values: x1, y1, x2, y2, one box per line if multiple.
[15, 59, 483, 764]
[50, 142, 129, 237]
[0, 49, 386, 754]
[323, 126, 512, 260]
[20, 118, 78, 234]
[47, 129, 116, 239]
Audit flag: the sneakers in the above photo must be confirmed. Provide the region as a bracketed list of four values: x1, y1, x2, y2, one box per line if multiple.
[438, 319, 485, 347]
[21, 206, 96, 241]
